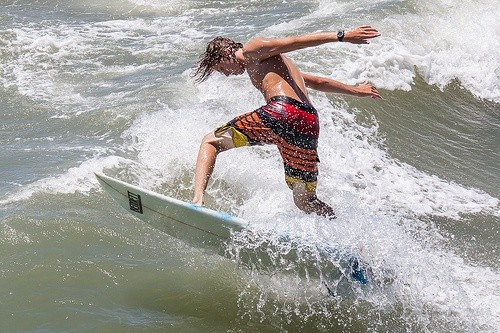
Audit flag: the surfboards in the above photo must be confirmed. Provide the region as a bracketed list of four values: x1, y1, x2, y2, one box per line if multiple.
[94, 170, 371, 284]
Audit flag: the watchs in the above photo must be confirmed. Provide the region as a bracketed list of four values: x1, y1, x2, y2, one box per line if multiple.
[337, 30, 346, 41]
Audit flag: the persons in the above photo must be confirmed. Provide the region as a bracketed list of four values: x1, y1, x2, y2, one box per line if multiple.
[190, 23, 383, 218]
